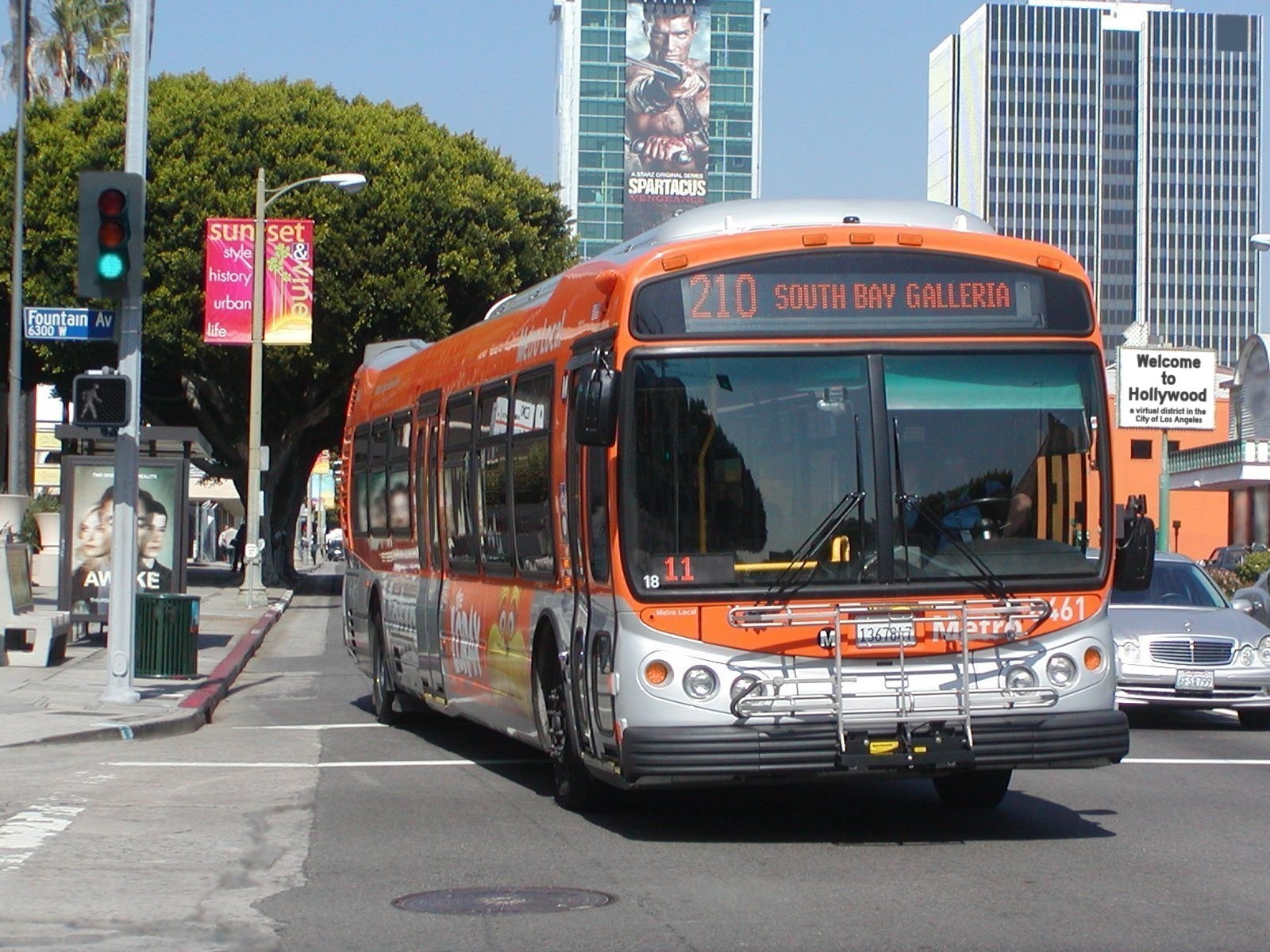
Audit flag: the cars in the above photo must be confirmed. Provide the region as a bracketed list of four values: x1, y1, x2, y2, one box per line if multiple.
[1198, 546, 1265, 575]
[1233, 568, 1269, 628]
[1082, 547, 1270, 733]
[327, 541, 345, 562]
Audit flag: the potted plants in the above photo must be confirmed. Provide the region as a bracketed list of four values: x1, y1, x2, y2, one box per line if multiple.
[21, 492, 59, 554]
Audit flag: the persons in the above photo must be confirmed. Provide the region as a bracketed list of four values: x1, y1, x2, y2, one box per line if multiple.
[218, 523, 247, 572]
[72, 484, 172, 614]
[891, 434, 1033, 553]
[624, 0, 709, 188]
[299, 534, 326, 565]
[25, 509, 43, 587]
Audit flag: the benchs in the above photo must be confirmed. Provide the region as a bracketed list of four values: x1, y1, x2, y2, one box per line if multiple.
[0, 544, 70, 666]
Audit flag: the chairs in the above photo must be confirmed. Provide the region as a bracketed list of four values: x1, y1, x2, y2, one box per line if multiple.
[1227, 552, 1234, 563]
[1113, 584, 1136, 602]
[1138, 583, 1180, 600]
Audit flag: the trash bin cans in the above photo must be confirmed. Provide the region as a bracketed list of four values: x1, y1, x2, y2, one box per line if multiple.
[135, 592, 200, 678]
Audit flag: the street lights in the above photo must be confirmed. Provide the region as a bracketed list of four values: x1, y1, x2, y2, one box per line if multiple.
[238, 166, 366, 605]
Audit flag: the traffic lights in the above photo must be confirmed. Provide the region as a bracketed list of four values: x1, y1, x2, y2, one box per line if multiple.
[77, 173, 145, 300]
[74, 375, 132, 427]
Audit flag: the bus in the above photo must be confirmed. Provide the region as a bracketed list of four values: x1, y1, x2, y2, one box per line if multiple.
[339, 199, 1156, 814]
